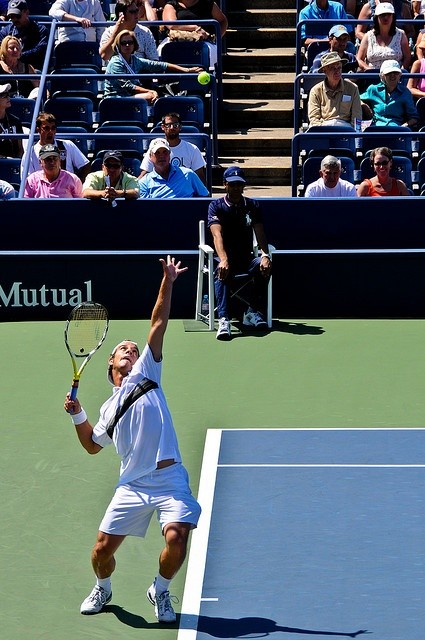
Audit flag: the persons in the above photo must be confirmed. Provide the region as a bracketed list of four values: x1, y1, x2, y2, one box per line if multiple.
[308, 52, 362, 130]
[24, 144, 83, 198]
[412, 0, 425, 19]
[356, 2, 411, 70]
[137, 112, 206, 184]
[162, 0, 228, 39]
[120, 0, 158, 28]
[415, 25, 425, 59]
[208, 166, 272, 340]
[357, 146, 411, 196]
[0, 83, 24, 159]
[355, 0, 416, 42]
[64, 256, 201, 623]
[406, 58, 425, 98]
[0, 35, 39, 97]
[311, 24, 358, 74]
[360, 60, 420, 127]
[105, 30, 203, 99]
[139, 138, 211, 198]
[299, 0, 353, 51]
[83, 150, 140, 198]
[0, 0, 48, 67]
[0, 179, 17, 200]
[49, 0, 109, 70]
[304, 155, 357, 197]
[99, 1, 160, 61]
[20, 112, 93, 182]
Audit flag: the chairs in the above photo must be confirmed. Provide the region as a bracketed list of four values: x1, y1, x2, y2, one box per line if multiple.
[92, 126, 146, 155]
[90, 158, 143, 179]
[195, 218, 277, 332]
[42, 96, 92, 125]
[307, 41, 356, 71]
[153, 96, 205, 127]
[98, 99, 148, 128]
[304, 127, 353, 154]
[161, 41, 210, 73]
[53, 127, 87, 157]
[171, 63, 208, 93]
[5, 98, 35, 128]
[0, 158, 21, 191]
[362, 126, 413, 155]
[48, 70, 98, 96]
[361, 157, 413, 188]
[54, 41, 103, 71]
[302, 157, 354, 189]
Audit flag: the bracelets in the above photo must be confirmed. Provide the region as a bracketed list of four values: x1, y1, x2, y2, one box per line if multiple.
[261, 253, 271, 258]
[120, 189, 128, 197]
[70, 407, 88, 425]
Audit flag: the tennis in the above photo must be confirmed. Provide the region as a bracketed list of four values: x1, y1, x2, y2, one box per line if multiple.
[197, 71, 210, 85]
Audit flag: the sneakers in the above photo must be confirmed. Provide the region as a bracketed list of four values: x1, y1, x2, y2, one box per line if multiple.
[80, 584, 112, 615]
[146, 582, 176, 623]
[216, 318, 231, 340]
[243, 311, 268, 329]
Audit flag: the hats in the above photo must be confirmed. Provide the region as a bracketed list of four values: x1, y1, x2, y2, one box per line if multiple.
[107, 341, 138, 386]
[39, 144, 60, 159]
[329, 25, 349, 38]
[7, 0, 27, 16]
[380, 60, 401, 74]
[149, 138, 171, 153]
[0, 83, 12, 93]
[223, 166, 246, 183]
[375, 3, 395, 15]
[104, 150, 124, 162]
[318, 51, 348, 73]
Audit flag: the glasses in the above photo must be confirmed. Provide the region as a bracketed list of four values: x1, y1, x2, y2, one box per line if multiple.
[104, 162, 121, 169]
[121, 40, 134, 46]
[164, 123, 180, 129]
[128, 7, 139, 14]
[41, 125, 56, 131]
[374, 160, 389, 166]
[0, 93, 10, 99]
